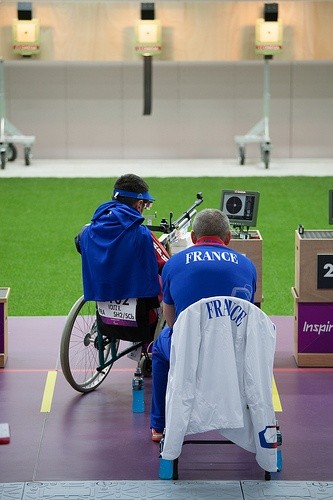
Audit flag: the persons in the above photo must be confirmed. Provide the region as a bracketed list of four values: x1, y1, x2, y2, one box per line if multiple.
[74, 174, 169, 304]
[150, 208, 256, 442]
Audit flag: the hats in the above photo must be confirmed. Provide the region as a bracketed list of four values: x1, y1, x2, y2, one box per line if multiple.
[112, 188, 157, 202]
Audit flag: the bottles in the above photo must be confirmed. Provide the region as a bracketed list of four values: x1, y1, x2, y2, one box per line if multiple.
[158, 428, 173, 480]
[131, 367, 145, 413]
[276, 420, 282, 472]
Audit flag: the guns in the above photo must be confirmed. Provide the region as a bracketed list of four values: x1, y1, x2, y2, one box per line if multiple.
[157, 192, 204, 249]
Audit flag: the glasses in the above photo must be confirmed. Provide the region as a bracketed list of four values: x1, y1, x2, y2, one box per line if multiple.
[143, 201, 153, 209]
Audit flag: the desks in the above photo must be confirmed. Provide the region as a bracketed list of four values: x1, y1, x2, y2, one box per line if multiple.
[292, 227, 333, 367]
[0, 287, 11, 368]
[225, 229, 265, 310]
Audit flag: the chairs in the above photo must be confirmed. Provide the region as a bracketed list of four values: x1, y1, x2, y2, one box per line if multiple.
[161, 294, 278, 483]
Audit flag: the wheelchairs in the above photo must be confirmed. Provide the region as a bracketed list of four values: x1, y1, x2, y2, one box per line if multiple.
[60, 234, 168, 395]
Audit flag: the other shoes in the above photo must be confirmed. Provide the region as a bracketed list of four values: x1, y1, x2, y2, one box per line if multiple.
[151, 427, 164, 441]
[141, 347, 151, 356]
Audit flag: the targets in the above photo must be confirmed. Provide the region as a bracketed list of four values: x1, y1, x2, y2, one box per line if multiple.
[223, 194, 246, 216]
